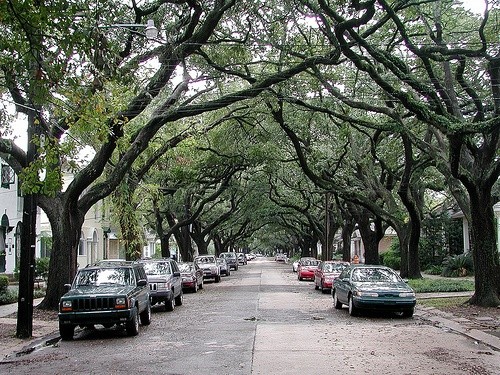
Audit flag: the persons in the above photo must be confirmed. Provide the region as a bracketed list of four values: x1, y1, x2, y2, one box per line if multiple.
[354, 252, 359, 264]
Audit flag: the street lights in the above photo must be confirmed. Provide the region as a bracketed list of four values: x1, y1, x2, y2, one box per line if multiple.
[23, 20, 158, 337]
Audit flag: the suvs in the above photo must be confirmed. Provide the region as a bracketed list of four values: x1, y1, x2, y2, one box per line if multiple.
[219, 252, 238, 270]
[193, 255, 220, 282]
[58, 259, 152, 340]
[136, 258, 183, 311]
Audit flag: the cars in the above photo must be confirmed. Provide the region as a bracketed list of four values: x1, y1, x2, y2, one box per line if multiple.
[216, 258, 230, 276]
[176, 261, 203, 292]
[297, 257, 322, 281]
[275, 254, 286, 263]
[331, 264, 416, 318]
[244, 253, 262, 261]
[315, 260, 351, 292]
[236, 252, 247, 265]
[292, 259, 298, 272]
